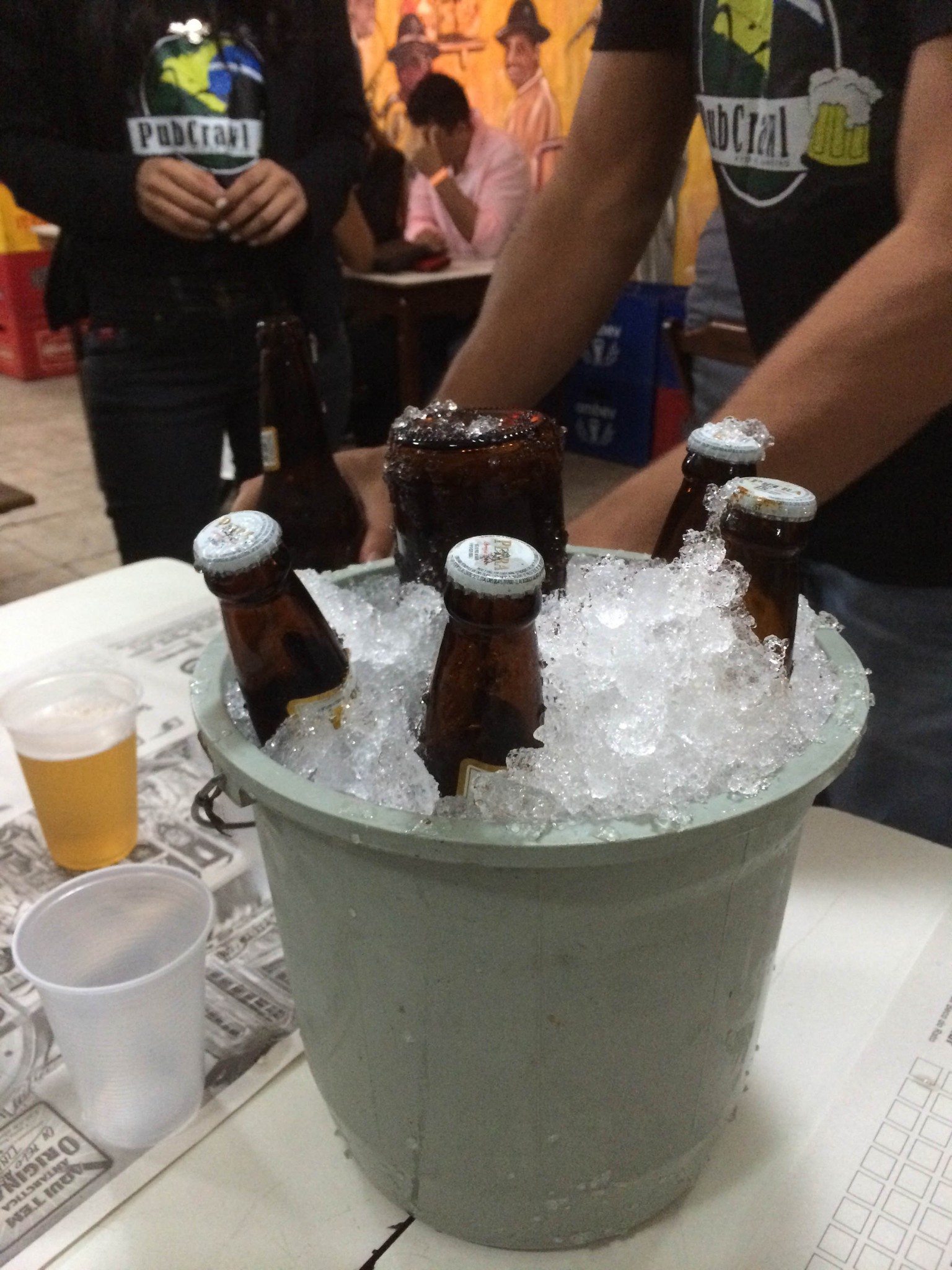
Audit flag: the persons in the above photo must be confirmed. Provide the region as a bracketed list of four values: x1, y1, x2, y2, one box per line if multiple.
[229, 0, 952, 850]
[1, 1, 367, 564]
[495, 0, 562, 191]
[335, 73, 532, 446]
[382, 12, 441, 152]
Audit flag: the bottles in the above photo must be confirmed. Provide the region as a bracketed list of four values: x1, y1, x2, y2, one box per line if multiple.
[380, 406, 568, 603]
[412, 533, 548, 807]
[709, 474, 820, 680]
[190, 507, 351, 752]
[648, 423, 775, 569]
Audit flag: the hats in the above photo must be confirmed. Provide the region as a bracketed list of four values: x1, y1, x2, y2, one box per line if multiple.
[494, 0, 550, 44]
[386, 12, 440, 62]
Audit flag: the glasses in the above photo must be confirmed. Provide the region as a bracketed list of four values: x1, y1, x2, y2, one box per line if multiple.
[395, 56, 422, 72]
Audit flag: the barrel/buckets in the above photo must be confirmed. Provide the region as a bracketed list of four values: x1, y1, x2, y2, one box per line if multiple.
[189, 542, 870, 1254]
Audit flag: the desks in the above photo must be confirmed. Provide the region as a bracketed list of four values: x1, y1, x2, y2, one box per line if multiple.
[1, 541, 952, 1268]
[344, 260, 496, 444]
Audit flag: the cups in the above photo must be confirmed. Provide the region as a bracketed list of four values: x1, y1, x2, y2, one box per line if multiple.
[10, 863, 215, 1151]
[0, 667, 150, 870]
